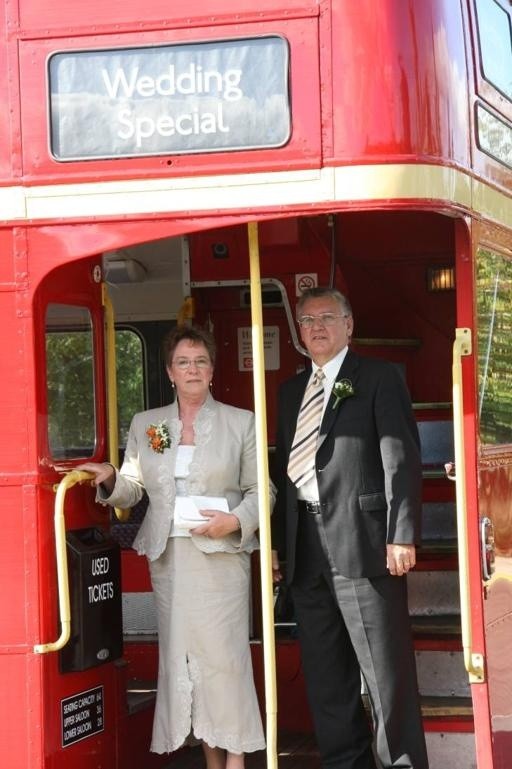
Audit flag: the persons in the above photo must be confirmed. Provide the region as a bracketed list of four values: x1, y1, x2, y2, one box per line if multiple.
[71, 319, 279, 769]
[257, 284, 433, 769]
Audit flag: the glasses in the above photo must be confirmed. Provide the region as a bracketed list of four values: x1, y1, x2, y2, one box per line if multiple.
[296, 311, 345, 329]
[174, 357, 209, 368]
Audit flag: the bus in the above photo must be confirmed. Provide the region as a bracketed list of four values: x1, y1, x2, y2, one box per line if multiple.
[2, 0, 512, 769]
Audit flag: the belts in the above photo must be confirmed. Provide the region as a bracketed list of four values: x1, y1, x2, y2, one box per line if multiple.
[297, 500, 322, 517]
